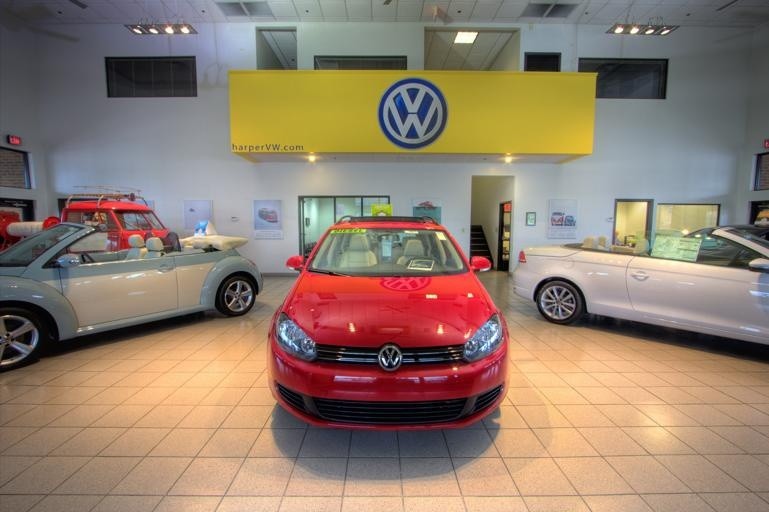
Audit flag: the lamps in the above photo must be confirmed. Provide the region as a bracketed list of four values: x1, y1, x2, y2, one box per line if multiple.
[123, 24, 198, 35]
[605, 24, 680, 37]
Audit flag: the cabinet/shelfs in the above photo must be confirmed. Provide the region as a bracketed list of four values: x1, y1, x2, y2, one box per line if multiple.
[503, 203, 511, 272]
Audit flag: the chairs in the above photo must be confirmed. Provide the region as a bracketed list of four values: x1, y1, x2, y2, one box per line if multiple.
[390, 235, 412, 262]
[124, 233, 145, 261]
[143, 237, 164, 258]
[338, 235, 376, 268]
[580, 236, 595, 250]
[396, 239, 431, 264]
[632, 239, 651, 258]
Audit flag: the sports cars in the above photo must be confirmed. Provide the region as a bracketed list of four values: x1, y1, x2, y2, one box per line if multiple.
[512, 220, 769, 350]
[0, 219, 264, 373]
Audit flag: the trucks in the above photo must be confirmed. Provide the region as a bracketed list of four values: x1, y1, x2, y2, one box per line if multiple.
[0, 193, 177, 258]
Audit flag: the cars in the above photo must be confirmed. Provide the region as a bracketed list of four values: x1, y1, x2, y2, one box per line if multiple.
[267, 215, 511, 439]
